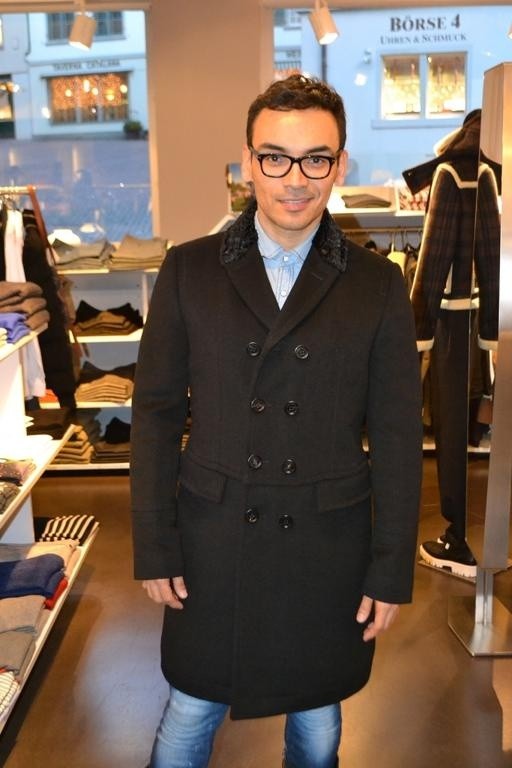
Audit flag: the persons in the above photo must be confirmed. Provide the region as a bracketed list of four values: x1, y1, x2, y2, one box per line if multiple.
[131, 74, 424, 767]
[408, 107, 482, 580]
[470, 152, 503, 445]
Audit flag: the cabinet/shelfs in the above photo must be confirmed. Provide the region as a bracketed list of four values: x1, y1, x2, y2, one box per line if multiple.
[0, 322, 100, 734]
[210, 207, 490, 454]
[39, 242, 158, 473]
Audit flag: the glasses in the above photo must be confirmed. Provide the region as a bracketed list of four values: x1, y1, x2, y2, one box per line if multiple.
[248, 145, 343, 179]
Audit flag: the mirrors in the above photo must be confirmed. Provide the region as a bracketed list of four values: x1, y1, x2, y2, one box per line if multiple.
[445, 61, 511, 661]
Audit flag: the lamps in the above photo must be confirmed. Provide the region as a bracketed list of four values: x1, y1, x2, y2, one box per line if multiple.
[68, 0, 97, 52]
[308, 0, 340, 46]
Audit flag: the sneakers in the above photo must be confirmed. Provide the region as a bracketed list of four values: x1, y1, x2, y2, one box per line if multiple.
[419, 533, 477, 577]
